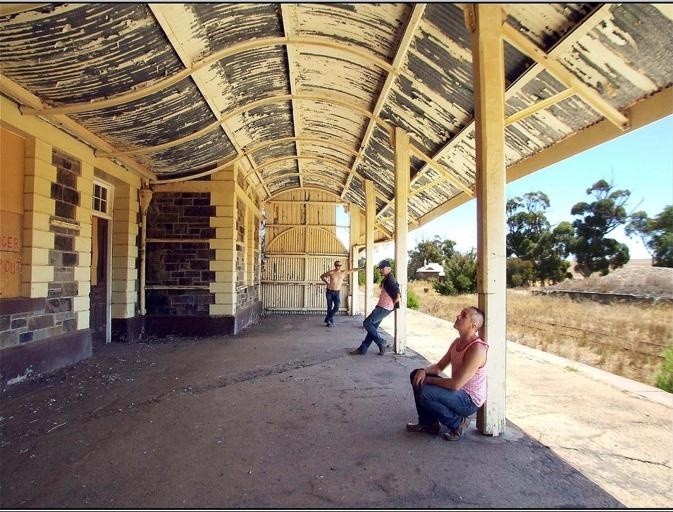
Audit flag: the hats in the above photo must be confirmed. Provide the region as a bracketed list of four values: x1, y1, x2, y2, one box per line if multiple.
[376, 260, 391, 268]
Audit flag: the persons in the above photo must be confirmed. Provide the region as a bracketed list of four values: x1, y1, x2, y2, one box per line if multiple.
[407, 306, 489, 440]
[349, 260, 401, 356]
[320, 260, 366, 327]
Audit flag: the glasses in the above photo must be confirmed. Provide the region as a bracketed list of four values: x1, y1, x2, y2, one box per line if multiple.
[336, 265, 342, 266]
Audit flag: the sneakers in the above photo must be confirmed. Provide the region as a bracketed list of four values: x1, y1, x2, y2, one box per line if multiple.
[379, 340, 386, 355]
[407, 421, 440, 434]
[444, 416, 470, 441]
[324, 318, 335, 327]
[350, 348, 366, 355]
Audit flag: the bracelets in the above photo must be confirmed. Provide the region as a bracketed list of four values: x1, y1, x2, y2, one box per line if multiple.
[419, 368, 426, 373]
